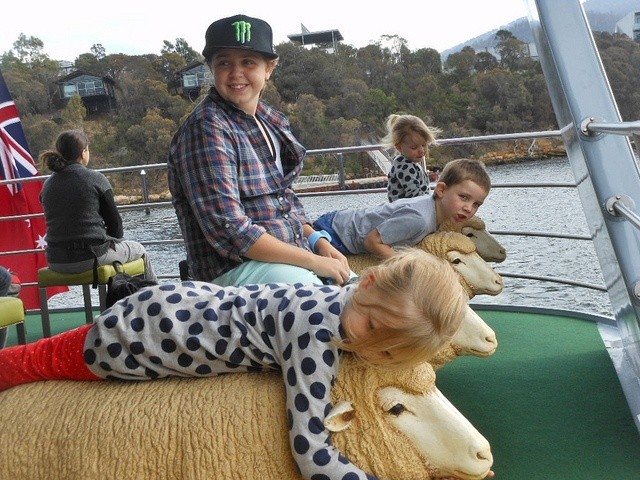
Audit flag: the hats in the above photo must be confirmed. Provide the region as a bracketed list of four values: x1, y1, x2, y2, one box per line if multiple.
[203, 15, 278, 60]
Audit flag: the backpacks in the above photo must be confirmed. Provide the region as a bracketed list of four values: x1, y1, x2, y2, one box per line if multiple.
[105, 262, 158, 312]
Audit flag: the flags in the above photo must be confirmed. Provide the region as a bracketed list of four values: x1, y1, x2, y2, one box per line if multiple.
[0, 174, 68, 310]
[1, 71, 39, 198]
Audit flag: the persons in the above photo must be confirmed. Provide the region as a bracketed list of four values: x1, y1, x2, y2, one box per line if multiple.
[38, 129, 159, 308]
[312, 160, 492, 264]
[166, 14, 354, 289]
[386, 113, 430, 205]
[0, 266, 21, 298]
[2, 250, 466, 477]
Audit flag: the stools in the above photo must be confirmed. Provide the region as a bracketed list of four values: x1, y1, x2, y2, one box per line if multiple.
[0, 296, 29, 345]
[37, 256, 145, 337]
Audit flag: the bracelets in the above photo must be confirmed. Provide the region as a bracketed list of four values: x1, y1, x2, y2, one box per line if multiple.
[307, 228, 333, 249]
